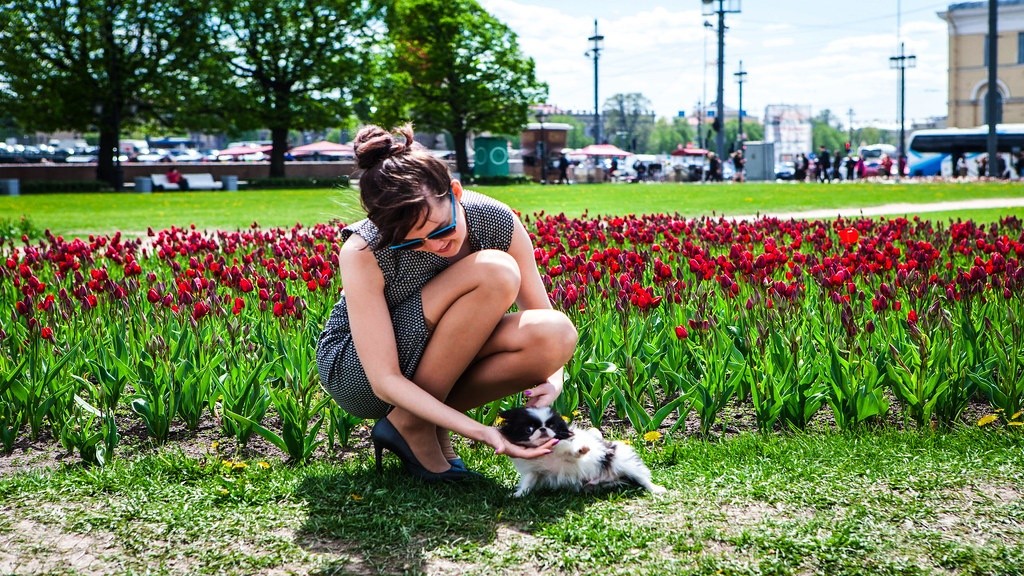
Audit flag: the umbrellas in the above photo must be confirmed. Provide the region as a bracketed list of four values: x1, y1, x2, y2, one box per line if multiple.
[571, 144, 633, 155]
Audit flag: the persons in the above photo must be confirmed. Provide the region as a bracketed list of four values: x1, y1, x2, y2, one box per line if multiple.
[316, 112, 578, 480]
[812, 152, 821, 182]
[878, 155, 893, 180]
[952, 145, 1024, 179]
[819, 146, 831, 184]
[844, 155, 857, 181]
[167, 167, 190, 192]
[212, 155, 222, 162]
[554, 151, 570, 185]
[285, 149, 340, 161]
[793, 153, 809, 173]
[855, 155, 867, 178]
[603, 158, 618, 181]
[633, 159, 646, 183]
[729, 150, 747, 184]
[229, 155, 240, 161]
[832, 149, 843, 183]
[125, 147, 133, 158]
[897, 155, 907, 177]
[665, 152, 723, 183]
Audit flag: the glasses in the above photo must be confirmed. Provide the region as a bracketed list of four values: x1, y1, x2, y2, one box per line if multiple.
[388, 186, 457, 251]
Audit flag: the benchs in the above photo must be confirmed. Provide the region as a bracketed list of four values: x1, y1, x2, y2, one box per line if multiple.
[151, 173, 223, 192]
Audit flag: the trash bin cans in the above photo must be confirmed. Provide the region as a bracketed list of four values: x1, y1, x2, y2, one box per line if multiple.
[589, 168, 603, 183]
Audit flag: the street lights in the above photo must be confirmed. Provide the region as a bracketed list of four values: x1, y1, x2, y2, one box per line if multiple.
[701, 0, 741, 179]
[889, 43, 915, 175]
[584, 20, 604, 163]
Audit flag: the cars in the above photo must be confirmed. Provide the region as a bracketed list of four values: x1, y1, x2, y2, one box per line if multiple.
[568, 154, 737, 180]
[774, 161, 796, 179]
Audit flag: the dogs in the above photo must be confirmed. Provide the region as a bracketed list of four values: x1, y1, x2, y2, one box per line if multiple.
[499, 406, 668, 500]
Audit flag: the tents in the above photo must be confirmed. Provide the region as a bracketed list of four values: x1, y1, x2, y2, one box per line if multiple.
[673, 148, 709, 156]
[286, 141, 355, 156]
[218, 146, 273, 154]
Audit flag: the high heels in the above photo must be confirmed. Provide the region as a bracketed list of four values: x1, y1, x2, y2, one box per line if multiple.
[371, 417, 472, 479]
[447, 456, 484, 478]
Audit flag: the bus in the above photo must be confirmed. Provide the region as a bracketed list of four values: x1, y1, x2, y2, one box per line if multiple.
[858, 143, 898, 174]
[906, 122, 1024, 181]
